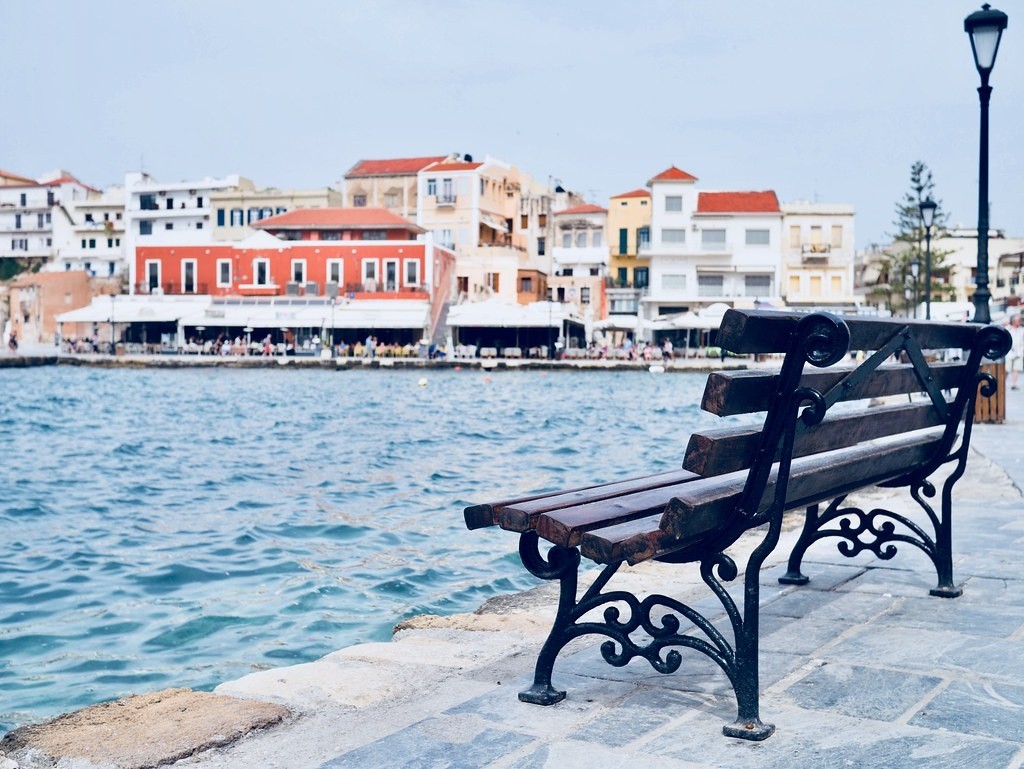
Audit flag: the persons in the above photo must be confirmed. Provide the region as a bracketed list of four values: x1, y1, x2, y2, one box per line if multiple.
[1003, 314, 1024, 391]
[9, 327, 19, 353]
[61, 333, 710, 363]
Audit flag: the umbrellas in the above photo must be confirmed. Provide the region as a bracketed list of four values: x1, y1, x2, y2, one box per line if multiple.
[591, 310, 725, 360]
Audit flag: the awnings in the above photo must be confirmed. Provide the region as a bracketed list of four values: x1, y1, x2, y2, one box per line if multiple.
[57, 293, 563, 328]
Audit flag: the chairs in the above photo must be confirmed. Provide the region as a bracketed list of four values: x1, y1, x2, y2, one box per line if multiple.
[337, 344, 418, 358]
[455, 345, 476, 359]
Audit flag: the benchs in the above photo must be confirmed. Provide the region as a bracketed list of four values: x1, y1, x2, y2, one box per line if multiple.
[464, 309, 1013, 742]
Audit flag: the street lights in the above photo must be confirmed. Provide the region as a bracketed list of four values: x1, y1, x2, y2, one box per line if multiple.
[918, 195, 937, 321]
[962, 5, 1010, 326]
